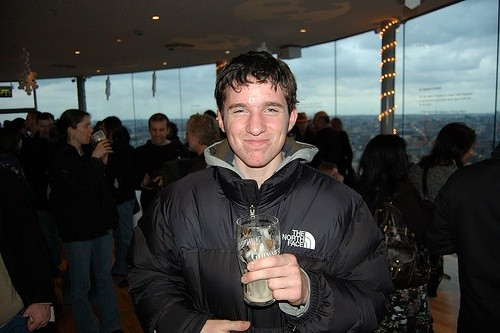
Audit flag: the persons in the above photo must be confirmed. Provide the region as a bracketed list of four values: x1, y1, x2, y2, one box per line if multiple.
[287, 111, 476, 333]
[0, 110, 227, 333]
[124, 51, 395, 333]
[422, 140, 500, 333]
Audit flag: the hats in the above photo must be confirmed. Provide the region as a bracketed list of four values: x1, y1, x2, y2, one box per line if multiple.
[296, 112, 309, 123]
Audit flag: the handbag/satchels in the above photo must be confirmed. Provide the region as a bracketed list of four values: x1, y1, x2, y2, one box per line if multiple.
[373, 202, 430, 289]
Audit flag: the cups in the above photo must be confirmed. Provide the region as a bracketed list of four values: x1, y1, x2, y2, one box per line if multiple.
[234, 214, 282, 307]
[91, 131, 106, 146]
